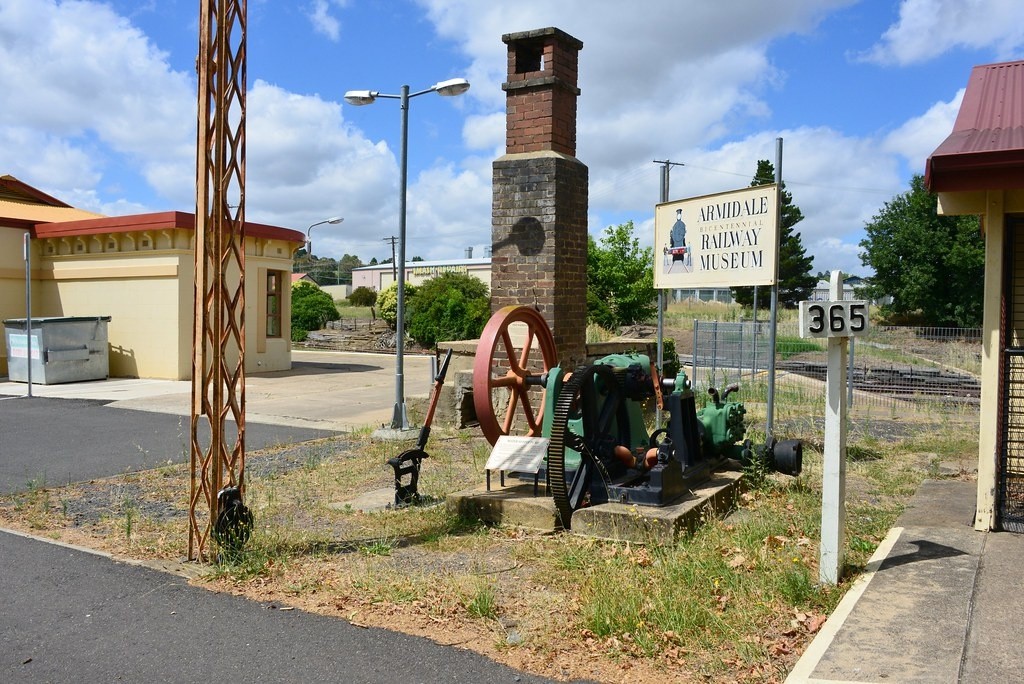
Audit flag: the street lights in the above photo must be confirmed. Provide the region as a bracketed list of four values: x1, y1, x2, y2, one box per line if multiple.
[338, 78, 472, 429]
[305, 216, 345, 262]
